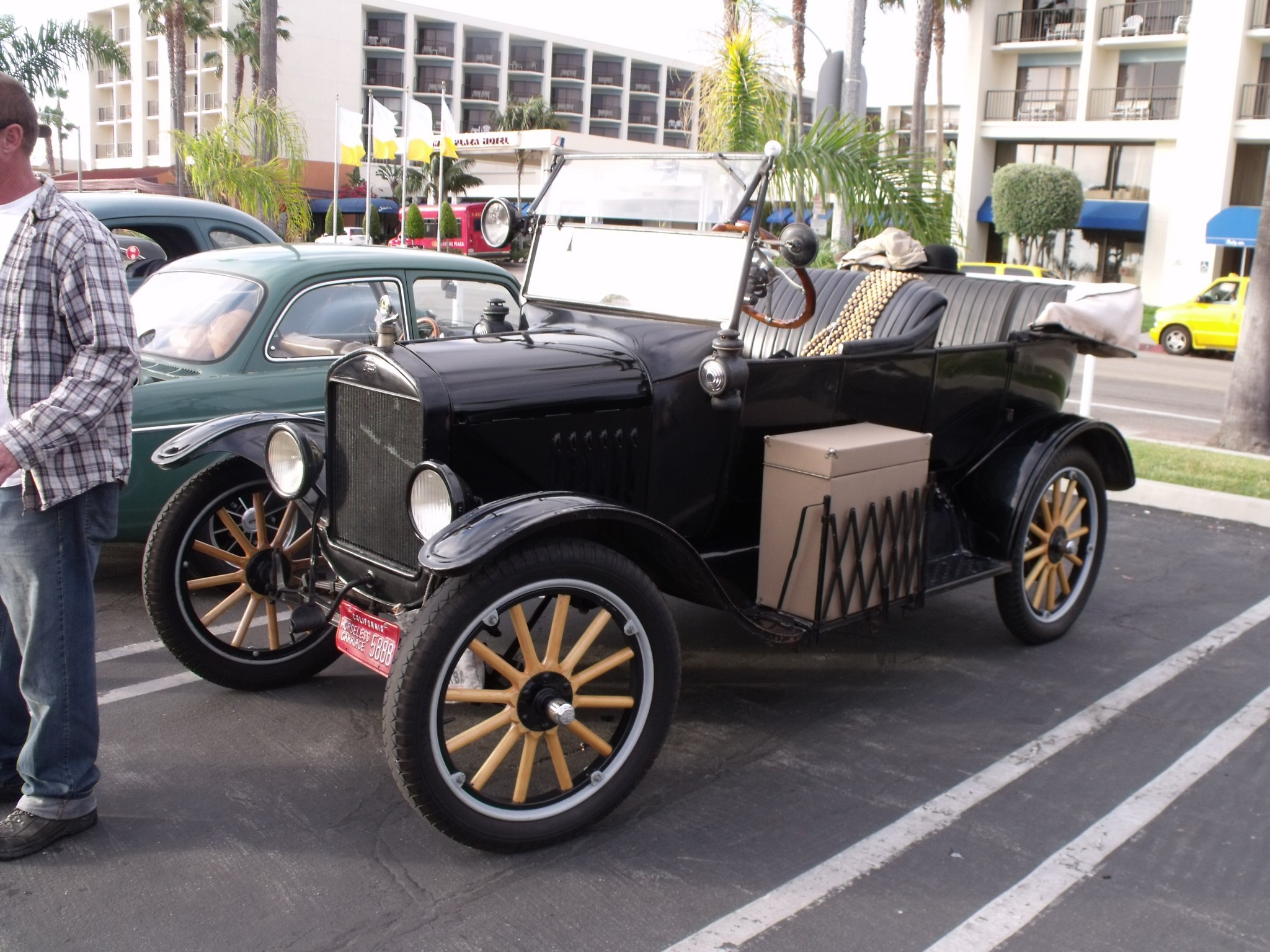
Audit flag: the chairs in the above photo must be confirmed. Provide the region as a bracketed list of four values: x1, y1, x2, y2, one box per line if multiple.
[471, 90, 491, 100]
[1045, 22, 1084, 40]
[1017, 100, 1060, 122]
[669, 90, 683, 98]
[557, 103, 575, 112]
[668, 120, 683, 130]
[1172, 14, 1190, 35]
[598, 76, 613, 86]
[428, 83, 444, 93]
[510, 60, 537, 72]
[635, 84, 650, 92]
[435, 120, 441, 130]
[476, 53, 493, 63]
[598, 109, 613, 119]
[1121, 15, 1143, 38]
[471, 125, 490, 133]
[642, 115, 652, 124]
[422, 45, 447, 56]
[1109, 99, 1151, 122]
[517, 97, 529, 105]
[366, 35, 392, 47]
[558, 69, 577, 78]
[368, 79, 376, 85]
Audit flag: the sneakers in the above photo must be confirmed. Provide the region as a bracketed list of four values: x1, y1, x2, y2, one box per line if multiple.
[0, 806, 100, 859]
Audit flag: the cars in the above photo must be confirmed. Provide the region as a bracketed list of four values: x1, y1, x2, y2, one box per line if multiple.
[1149, 273, 1249, 356]
[59, 134, 1141, 854]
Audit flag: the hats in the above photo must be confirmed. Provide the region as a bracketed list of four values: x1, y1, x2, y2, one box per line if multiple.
[919, 245, 964, 276]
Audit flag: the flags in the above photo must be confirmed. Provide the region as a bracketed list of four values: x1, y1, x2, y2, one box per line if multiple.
[338, 108, 365, 166]
[373, 98, 398, 160]
[407, 98, 435, 164]
[441, 100, 458, 158]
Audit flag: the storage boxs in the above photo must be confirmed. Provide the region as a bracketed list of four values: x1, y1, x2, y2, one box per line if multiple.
[757, 423, 933, 621]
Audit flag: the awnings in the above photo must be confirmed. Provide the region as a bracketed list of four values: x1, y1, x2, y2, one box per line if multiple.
[1074, 201, 1149, 230]
[1206, 205, 1262, 246]
[809, 209, 832, 225]
[309, 198, 399, 214]
[861, 210, 906, 228]
[740, 206, 755, 221]
[767, 208, 796, 223]
[976, 196, 993, 223]
[787, 208, 813, 225]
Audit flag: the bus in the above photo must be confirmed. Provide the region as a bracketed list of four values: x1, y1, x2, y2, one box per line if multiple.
[388, 202, 513, 259]
[307, 197, 400, 242]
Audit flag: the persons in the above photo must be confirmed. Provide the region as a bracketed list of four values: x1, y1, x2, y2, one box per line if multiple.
[368, 64, 375, 84]
[0, 75, 139, 858]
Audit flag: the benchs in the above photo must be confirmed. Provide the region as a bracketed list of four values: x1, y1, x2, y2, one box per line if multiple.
[737, 268, 948, 358]
[914, 273, 1077, 347]
